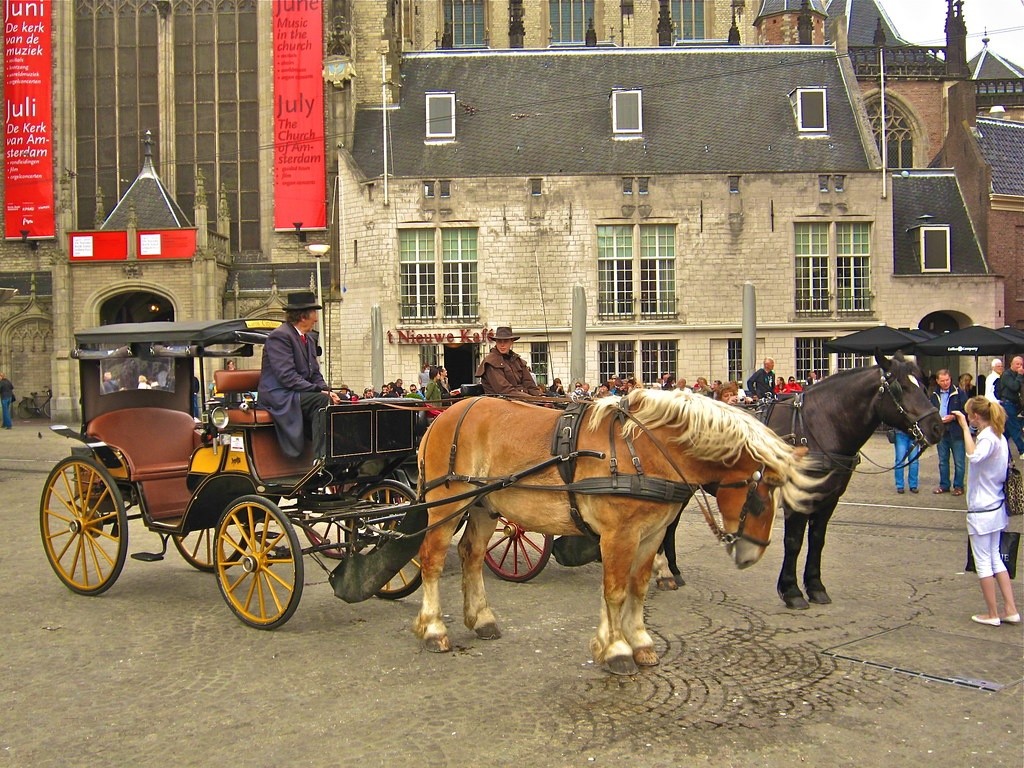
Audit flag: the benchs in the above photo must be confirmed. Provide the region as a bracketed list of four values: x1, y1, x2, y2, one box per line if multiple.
[75, 407, 206, 520]
[215, 369, 314, 479]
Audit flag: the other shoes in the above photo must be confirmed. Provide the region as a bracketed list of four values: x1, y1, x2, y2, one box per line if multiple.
[1020, 453, 1024, 459]
[910, 488, 918, 493]
[1000, 613, 1020, 623]
[898, 488, 904, 494]
[972, 616, 1000, 627]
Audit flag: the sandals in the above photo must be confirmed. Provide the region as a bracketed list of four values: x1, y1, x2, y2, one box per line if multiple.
[933, 487, 950, 494]
[952, 487, 964, 496]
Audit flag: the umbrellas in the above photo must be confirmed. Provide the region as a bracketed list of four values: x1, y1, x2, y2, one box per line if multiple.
[826, 325, 1024, 412]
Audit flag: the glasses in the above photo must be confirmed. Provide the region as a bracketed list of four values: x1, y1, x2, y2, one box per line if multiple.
[411, 389, 415, 392]
[575, 385, 580, 388]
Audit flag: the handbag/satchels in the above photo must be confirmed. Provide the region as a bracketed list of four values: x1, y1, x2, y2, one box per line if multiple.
[993, 378, 1001, 400]
[11, 393, 16, 402]
[965, 532, 1020, 579]
[1003, 445, 1024, 516]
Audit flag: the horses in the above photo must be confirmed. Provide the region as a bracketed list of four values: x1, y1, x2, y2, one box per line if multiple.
[653, 345, 946, 610]
[416, 387, 834, 678]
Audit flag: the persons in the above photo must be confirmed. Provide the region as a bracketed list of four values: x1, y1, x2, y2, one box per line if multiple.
[0, 372, 14, 430]
[339, 364, 454, 409]
[527, 367, 821, 412]
[958, 373, 976, 399]
[888, 421, 922, 495]
[100, 360, 199, 420]
[209, 360, 257, 402]
[257, 292, 349, 470]
[747, 357, 775, 399]
[931, 369, 968, 496]
[997, 356, 1024, 459]
[951, 395, 1020, 626]
[975, 375, 986, 396]
[984, 359, 1004, 405]
[480, 327, 550, 408]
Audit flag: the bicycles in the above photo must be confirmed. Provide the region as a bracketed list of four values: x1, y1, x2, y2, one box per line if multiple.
[18, 386, 52, 418]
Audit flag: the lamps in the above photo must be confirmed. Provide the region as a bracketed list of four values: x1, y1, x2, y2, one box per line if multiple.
[440, 180, 451, 198]
[833, 173, 847, 192]
[818, 174, 829, 192]
[727, 175, 740, 192]
[423, 179, 434, 198]
[531, 177, 543, 196]
[622, 175, 632, 197]
[20, 229, 37, 249]
[638, 176, 649, 194]
[293, 223, 306, 242]
[146, 295, 159, 313]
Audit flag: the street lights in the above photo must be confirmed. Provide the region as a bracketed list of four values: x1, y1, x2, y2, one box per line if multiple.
[304, 244, 333, 386]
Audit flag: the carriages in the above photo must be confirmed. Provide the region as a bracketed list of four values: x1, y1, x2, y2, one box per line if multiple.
[298, 351, 948, 612]
[39, 307, 833, 675]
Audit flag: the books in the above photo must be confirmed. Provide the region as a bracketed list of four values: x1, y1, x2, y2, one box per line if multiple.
[451, 388, 462, 395]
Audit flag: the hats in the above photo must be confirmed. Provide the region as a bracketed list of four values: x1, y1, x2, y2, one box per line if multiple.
[488, 327, 520, 341]
[282, 291, 323, 310]
